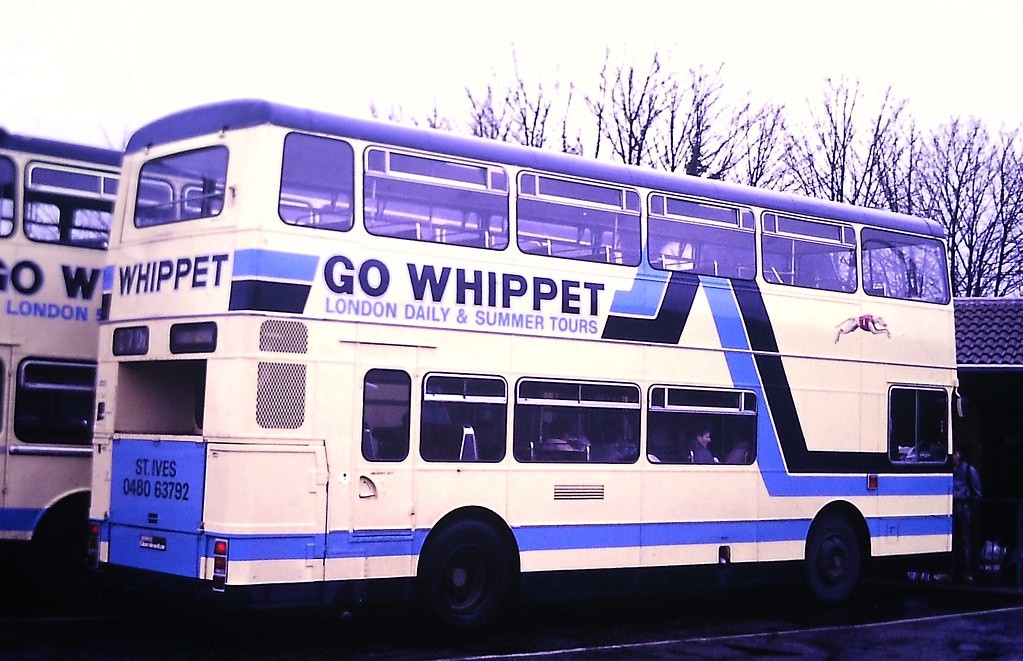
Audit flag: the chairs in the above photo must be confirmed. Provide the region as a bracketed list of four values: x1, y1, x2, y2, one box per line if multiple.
[366, 424, 752, 466]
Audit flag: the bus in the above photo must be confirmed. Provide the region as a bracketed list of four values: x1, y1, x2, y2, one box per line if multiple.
[0, 127, 127, 623]
[91, 99, 963, 621]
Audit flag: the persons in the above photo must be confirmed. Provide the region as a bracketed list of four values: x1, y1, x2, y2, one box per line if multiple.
[371, 388, 667, 462]
[936, 448, 982, 587]
[671, 425, 721, 466]
